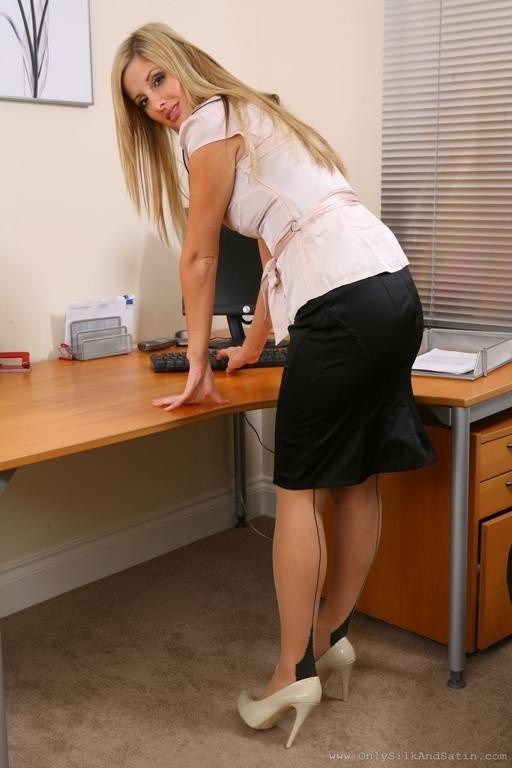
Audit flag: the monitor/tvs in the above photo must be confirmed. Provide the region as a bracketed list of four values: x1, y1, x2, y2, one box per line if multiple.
[182, 205, 290, 351]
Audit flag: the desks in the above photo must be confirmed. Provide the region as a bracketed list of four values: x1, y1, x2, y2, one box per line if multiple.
[2, 326, 511, 687]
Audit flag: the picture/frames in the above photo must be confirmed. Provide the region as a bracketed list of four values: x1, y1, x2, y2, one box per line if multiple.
[0, 0, 94, 107]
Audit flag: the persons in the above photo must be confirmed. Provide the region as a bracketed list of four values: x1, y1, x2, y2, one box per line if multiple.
[109, 21, 437, 747]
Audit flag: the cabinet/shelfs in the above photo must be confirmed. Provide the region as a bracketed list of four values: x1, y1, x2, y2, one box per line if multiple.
[316, 415, 510, 656]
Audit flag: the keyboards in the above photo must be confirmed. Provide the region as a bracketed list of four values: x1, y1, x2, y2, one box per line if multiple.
[149, 349, 289, 374]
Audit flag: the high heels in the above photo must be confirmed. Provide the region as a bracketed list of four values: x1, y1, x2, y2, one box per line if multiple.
[238, 636, 357, 749]
